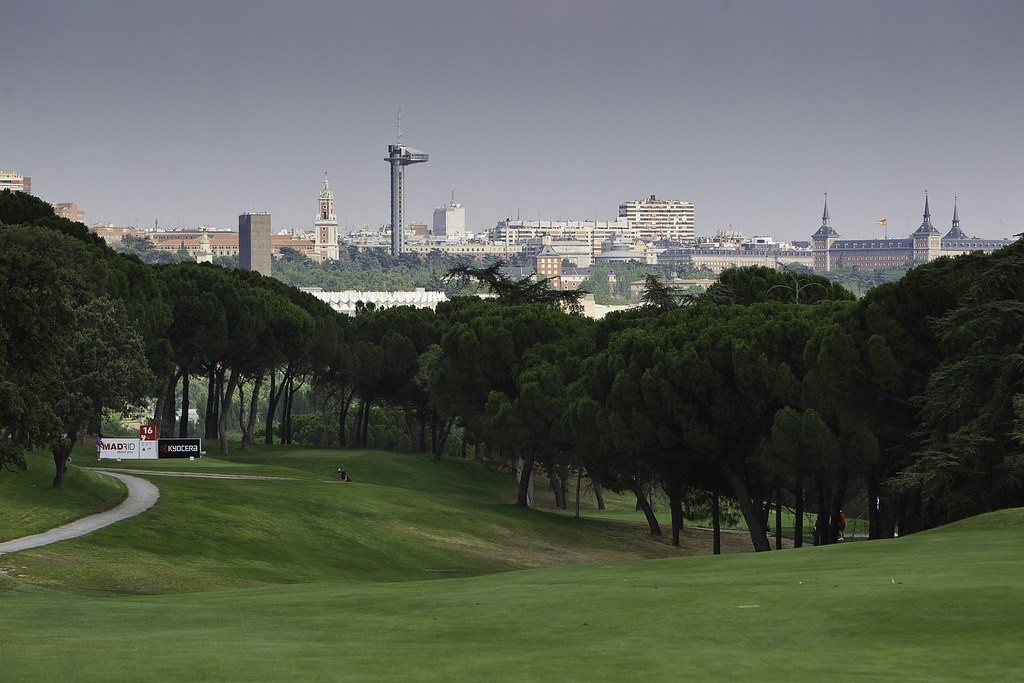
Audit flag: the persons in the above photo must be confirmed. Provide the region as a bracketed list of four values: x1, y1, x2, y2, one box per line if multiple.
[839, 510, 845, 540]
[96, 434, 104, 461]
[338, 467, 352, 482]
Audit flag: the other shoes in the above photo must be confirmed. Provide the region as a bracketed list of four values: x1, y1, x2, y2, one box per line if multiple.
[838, 538, 844, 540]
